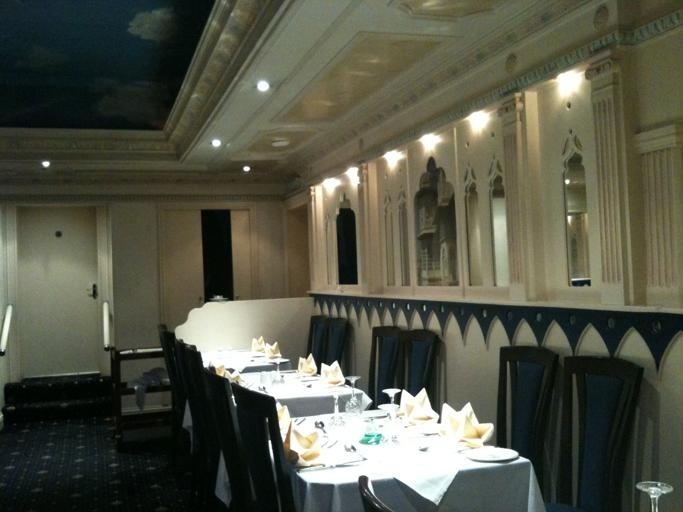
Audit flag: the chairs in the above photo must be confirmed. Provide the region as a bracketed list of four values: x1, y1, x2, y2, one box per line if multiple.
[154, 324, 206, 476]
[557, 354, 647, 510]
[305, 313, 355, 391]
[491, 344, 557, 510]
[230, 382, 297, 510]
[366, 324, 441, 421]
[203, 367, 256, 510]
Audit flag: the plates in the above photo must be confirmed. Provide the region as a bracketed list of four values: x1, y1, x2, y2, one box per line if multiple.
[466, 446, 520, 463]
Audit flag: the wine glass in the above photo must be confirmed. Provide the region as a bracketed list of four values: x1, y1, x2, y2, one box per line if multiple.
[377, 404, 401, 447]
[344, 375, 364, 419]
[269, 360, 287, 385]
[382, 388, 401, 423]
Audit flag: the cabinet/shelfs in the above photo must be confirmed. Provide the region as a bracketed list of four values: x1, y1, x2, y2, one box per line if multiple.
[103, 342, 174, 448]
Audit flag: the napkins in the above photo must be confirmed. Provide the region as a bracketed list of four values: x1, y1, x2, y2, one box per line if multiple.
[275, 400, 291, 430]
[396, 386, 441, 423]
[320, 359, 346, 388]
[265, 341, 282, 361]
[281, 418, 323, 468]
[297, 353, 318, 379]
[252, 336, 267, 354]
[208, 359, 225, 378]
[224, 370, 240, 384]
[441, 401, 496, 450]
